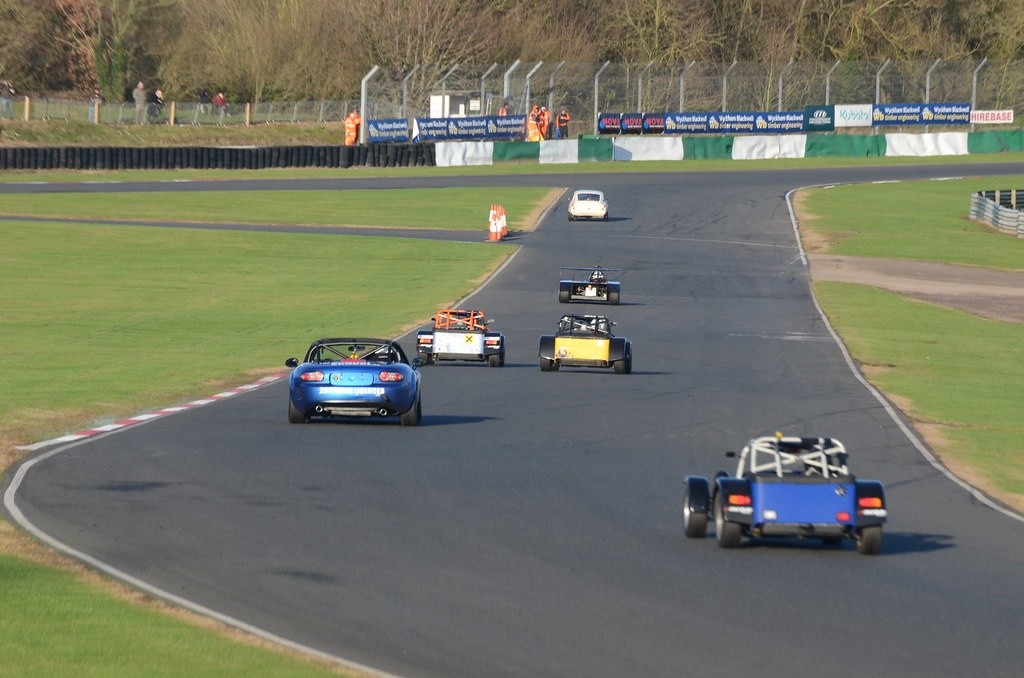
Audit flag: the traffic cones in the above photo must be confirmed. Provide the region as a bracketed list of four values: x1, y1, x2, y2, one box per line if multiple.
[484, 202, 509, 242]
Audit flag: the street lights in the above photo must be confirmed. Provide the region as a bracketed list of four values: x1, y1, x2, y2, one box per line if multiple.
[680, 59, 697, 113]
[875, 58, 891, 135]
[777, 61, 794, 112]
[403, 63, 420, 118]
[481, 62, 499, 116]
[549, 60, 566, 120]
[722, 60, 738, 112]
[593, 60, 611, 135]
[504, 59, 521, 106]
[636, 60, 655, 113]
[440, 63, 458, 117]
[358, 65, 379, 144]
[825, 60, 841, 134]
[969, 56, 989, 131]
[925, 56, 941, 133]
[525, 60, 545, 116]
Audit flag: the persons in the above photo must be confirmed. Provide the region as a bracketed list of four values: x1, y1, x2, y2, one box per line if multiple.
[352, 109, 360, 144]
[345, 113, 356, 145]
[556, 109, 570, 139]
[529, 105, 539, 121]
[498, 102, 508, 116]
[213, 92, 226, 126]
[540, 107, 550, 139]
[146, 89, 164, 123]
[133, 81, 147, 125]
[194, 91, 210, 125]
[88, 88, 103, 124]
[2, 82, 16, 121]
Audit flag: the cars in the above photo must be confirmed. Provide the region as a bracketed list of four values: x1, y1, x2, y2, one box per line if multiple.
[284, 337, 426, 427]
[682, 431, 887, 555]
[557, 266, 622, 305]
[538, 314, 631, 374]
[568, 188, 610, 222]
[415, 309, 505, 367]
